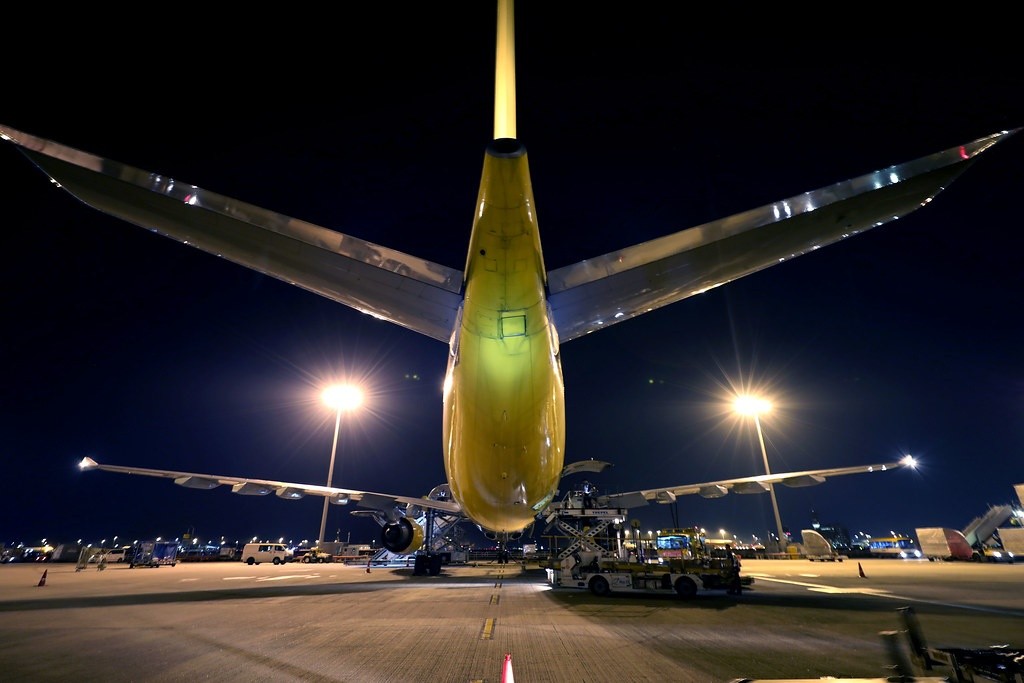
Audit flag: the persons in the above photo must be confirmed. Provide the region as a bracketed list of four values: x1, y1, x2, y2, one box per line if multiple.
[725, 544, 742, 596]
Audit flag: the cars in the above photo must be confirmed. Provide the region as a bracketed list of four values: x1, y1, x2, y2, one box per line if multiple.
[985, 547, 1016, 564]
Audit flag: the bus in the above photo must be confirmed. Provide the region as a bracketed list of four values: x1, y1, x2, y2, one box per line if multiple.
[869, 537, 918, 558]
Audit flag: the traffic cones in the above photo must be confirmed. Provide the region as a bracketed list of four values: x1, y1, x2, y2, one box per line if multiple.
[500, 653, 515, 683]
[32, 569, 48, 587]
[858, 562, 867, 578]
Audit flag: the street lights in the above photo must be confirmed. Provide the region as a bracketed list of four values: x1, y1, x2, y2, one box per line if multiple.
[318, 381, 363, 553]
[732, 393, 787, 553]
[719, 529, 726, 540]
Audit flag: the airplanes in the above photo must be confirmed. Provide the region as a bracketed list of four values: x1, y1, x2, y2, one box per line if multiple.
[0, 0, 1024, 580]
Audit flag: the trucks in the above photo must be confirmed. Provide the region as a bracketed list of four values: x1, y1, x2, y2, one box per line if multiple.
[649, 526, 706, 563]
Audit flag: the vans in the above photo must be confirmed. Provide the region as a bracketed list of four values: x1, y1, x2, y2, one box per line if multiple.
[242, 544, 294, 565]
[93, 549, 126, 564]
